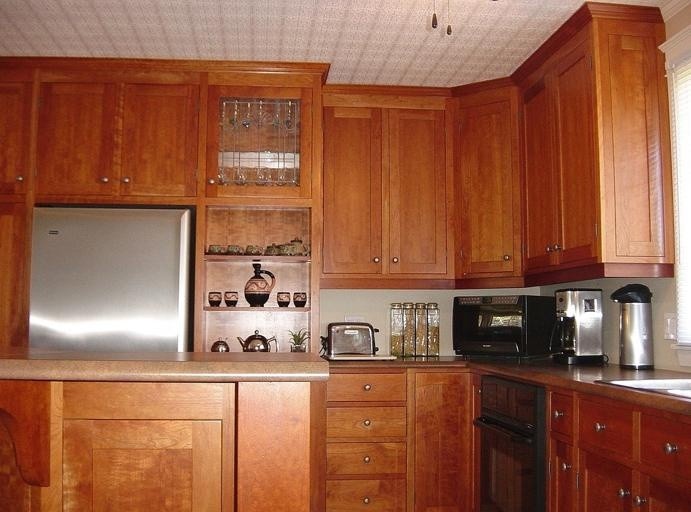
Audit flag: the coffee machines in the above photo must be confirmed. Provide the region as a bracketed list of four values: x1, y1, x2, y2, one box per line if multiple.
[545, 288, 606, 366]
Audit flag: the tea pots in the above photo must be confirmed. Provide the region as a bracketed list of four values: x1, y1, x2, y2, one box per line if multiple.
[245, 264, 276, 307]
[211, 337, 230, 352]
[290, 237, 312, 256]
[236, 329, 280, 353]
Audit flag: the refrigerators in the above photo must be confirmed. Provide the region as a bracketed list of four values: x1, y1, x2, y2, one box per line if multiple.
[30, 206, 193, 362]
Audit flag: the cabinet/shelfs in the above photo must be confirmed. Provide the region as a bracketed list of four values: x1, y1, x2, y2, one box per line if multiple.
[28, 56, 193, 198]
[453, 76, 524, 281]
[326, 370, 408, 512]
[322, 96, 448, 279]
[511, 0, 677, 287]
[61, 381, 237, 512]
[577, 384, 689, 511]
[0, 53, 33, 358]
[548, 388, 579, 511]
[192, 60, 326, 191]
[199, 190, 320, 363]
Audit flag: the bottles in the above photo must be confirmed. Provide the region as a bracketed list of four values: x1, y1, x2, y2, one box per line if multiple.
[389, 302, 441, 357]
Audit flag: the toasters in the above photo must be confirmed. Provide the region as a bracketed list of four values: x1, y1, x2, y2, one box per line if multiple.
[326, 321, 380, 357]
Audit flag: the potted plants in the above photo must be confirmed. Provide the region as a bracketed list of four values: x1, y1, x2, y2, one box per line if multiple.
[285, 328, 310, 353]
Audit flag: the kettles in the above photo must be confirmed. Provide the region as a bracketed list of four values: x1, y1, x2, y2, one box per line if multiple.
[612, 284, 657, 370]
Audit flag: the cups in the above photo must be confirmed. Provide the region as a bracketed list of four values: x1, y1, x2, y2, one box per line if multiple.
[211, 242, 294, 255]
[294, 292, 307, 307]
[208, 290, 222, 307]
[278, 292, 292, 307]
[224, 290, 239, 308]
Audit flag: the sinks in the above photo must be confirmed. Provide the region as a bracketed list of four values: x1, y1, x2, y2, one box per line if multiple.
[593, 378, 691, 390]
[650, 390, 691, 402]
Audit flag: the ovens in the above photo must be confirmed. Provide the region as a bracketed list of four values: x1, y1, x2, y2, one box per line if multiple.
[468, 373, 547, 512]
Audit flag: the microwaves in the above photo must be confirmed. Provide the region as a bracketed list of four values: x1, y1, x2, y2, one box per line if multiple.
[452, 294, 554, 363]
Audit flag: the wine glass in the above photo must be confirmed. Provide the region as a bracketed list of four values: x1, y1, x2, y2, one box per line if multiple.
[218, 165, 300, 186]
